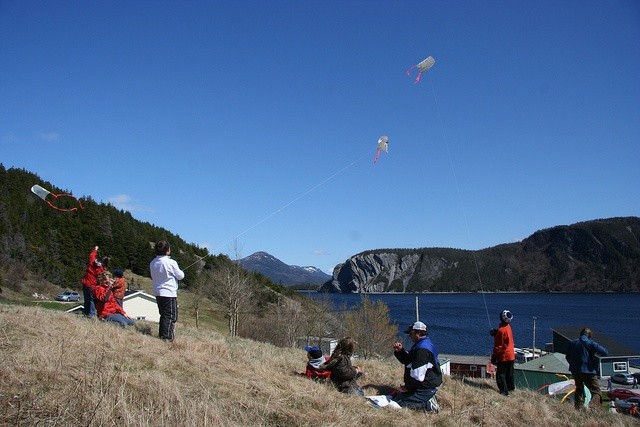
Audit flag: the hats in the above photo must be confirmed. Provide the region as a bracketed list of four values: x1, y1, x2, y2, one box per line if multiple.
[403, 322, 427, 333]
[113, 269, 123, 276]
[305, 346, 322, 358]
[502, 310, 513, 323]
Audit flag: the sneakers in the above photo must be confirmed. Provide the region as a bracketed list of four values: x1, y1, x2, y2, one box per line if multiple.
[429, 395, 440, 413]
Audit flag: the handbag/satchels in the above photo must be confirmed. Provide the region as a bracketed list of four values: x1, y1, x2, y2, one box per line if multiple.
[588, 355, 599, 371]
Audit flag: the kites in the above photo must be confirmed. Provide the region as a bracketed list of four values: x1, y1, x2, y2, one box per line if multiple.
[372, 135, 390, 168]
[406, 56, 435, 84]
[31, 184, 85, 211]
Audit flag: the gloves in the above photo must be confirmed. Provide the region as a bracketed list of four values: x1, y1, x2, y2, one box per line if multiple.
[490, 329, 498, 336]
[491, 355, 498, 365]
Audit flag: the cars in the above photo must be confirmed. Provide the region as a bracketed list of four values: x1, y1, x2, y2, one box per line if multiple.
[55, 291, 82, 302]
[610, 373, 638, 385]
[606, 388, 640, 401]
[631, 372, 640, 385]
[614, 397, 640, 408]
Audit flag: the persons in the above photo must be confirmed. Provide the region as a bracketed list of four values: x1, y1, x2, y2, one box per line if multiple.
[305, 346, 332, 379]
[320, 337, 364, 397]
[565, 328, 607, 410]
[82, 245, 110, 317]
[632, 376, 638, 389]
[92, 271, 136, 327]
[111, 268, 126, 310]
[393, 322, 442, 414]
[607, 378, 613, 390]
[489, 310, 515, 396]
[150, 240, 185, 341]
